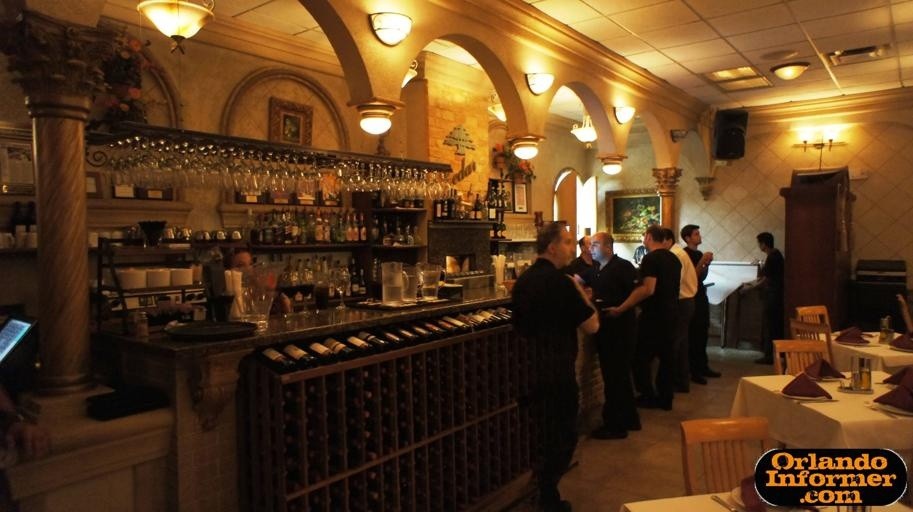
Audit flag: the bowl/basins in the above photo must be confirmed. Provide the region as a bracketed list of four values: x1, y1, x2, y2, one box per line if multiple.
[195, 229, 242, 240]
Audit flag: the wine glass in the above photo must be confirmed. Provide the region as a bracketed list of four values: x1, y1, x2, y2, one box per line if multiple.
[291, 150, 451, 201]
[109, 137, 289, 193]
[404, 223, 424, 246]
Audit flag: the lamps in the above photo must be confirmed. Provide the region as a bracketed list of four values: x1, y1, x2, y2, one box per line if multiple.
[769, 61, 811, 80]
[795, 124, 847, 151]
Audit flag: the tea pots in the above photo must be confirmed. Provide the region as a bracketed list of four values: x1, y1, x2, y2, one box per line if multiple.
[420, 264, 446, 302]
[379, 261, 409, 305]
[160, 225, 193, 241]
[398, 264, 424, 304]
[228, 259, 289, 330]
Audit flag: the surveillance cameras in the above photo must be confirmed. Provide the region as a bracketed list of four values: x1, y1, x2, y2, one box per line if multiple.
[672, 129, 688, 140]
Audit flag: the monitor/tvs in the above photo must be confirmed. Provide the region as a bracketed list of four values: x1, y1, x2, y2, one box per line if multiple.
[0, 315, 39, 378]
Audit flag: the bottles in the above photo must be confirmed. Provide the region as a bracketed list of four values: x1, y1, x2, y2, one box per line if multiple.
[246, 205, 330, 244]
[330, 211, 403, 247]
[134, 309, 150, 343]
[488, 212, 509, 239]
[851, 355, 871, 393]
[14, 224, 38, 250]
[430, 186, 508, 220]
[328, 258, 370, 298]
[283, 256, 329, 287]
[381, 300, 512, 349]
[878, 317, 893, 342]
[263, 330, 390, 375]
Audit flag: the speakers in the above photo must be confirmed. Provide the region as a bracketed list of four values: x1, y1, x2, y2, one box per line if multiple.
[712, 108, 748, 160]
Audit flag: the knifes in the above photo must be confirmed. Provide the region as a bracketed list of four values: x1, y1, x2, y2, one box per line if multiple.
[711, 495, 738, 512]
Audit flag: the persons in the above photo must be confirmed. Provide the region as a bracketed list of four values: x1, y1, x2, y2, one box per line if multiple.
[569, 224, 722, 441]
[754, 232, 785, 368]
[509, 219, 599, 511]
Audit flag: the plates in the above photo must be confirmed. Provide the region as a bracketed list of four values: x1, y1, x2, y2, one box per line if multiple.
[835, 338, 912, 354]
[776, 392, 835, 401]
[730, 484, 746, 508]
[802, 371, 849, 381]
[863, 398, 911, 418]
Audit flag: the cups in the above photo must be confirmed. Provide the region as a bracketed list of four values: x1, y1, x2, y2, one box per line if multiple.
[0, 232, 14, 249]
[509, 222, 538, 242]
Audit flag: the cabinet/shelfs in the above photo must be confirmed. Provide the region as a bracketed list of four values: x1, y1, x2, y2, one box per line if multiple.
[89, 241, 369, 394]
[352, 190, 426, 300]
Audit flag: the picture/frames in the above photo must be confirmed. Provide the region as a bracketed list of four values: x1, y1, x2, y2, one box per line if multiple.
[605, 188, 662, 243]
[512, 182, 528, 214]
[85, 171, 104, 198]
[267, 96, 314, 149]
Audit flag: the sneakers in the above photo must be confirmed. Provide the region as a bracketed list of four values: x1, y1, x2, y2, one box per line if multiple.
[591, 416, 642, 439]
[677, 380, 690, 392]
[540, 500, 571, 512]
[756, 357, 773, 364]
[635, 392, 673, 410]
[690, 363, 720, 384]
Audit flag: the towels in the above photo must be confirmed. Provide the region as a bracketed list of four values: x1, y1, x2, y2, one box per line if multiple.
[739, 475, 819, 512]
[782, 359, 913, 413]
[835, 325, 913, 352]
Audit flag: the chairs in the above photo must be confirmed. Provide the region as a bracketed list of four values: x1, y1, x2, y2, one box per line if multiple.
[796, 305, 831, 333]
[773, 340, 825, 375]
[790, 318, 835, 369]
[679, 416, 773, 496]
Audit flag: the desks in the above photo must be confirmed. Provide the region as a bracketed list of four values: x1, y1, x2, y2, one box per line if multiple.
[730, 370, 913, 502]
[620, 492, 913, 512]
[812, 331, 913, 375]
